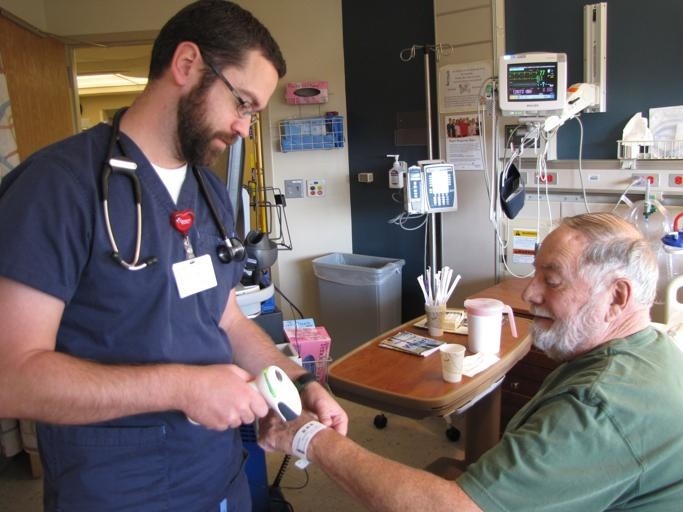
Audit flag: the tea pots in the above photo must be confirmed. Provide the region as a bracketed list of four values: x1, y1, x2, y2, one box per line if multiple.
[463, 297, 518, 354]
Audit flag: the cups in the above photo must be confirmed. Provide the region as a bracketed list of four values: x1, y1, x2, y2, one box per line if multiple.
[439, 344, 466, 383]
[424, 302, 446, 336]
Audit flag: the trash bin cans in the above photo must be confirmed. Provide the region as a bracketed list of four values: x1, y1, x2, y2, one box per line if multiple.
[312, 251, 406, 366]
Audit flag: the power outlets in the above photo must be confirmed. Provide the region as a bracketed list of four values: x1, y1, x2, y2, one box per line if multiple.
[287, 180, 301, 198]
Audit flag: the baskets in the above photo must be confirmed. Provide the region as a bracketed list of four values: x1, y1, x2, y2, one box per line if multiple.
[279, 115, 346, 153]
[302, 356, 333, 385]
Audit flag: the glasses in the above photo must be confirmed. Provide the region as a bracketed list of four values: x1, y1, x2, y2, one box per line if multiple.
[199, 56, 260, 127]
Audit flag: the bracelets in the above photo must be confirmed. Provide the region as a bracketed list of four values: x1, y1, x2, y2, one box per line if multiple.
[289, 370, 319, 397]
[290, 418, 330, 472]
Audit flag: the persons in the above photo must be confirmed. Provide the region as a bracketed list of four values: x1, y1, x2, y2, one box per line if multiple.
[2, 1, 351, 511]
[253, 209, 682, 511]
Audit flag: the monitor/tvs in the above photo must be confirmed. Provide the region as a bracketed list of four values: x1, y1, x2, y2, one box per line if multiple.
[498, 51, 568, 117]
